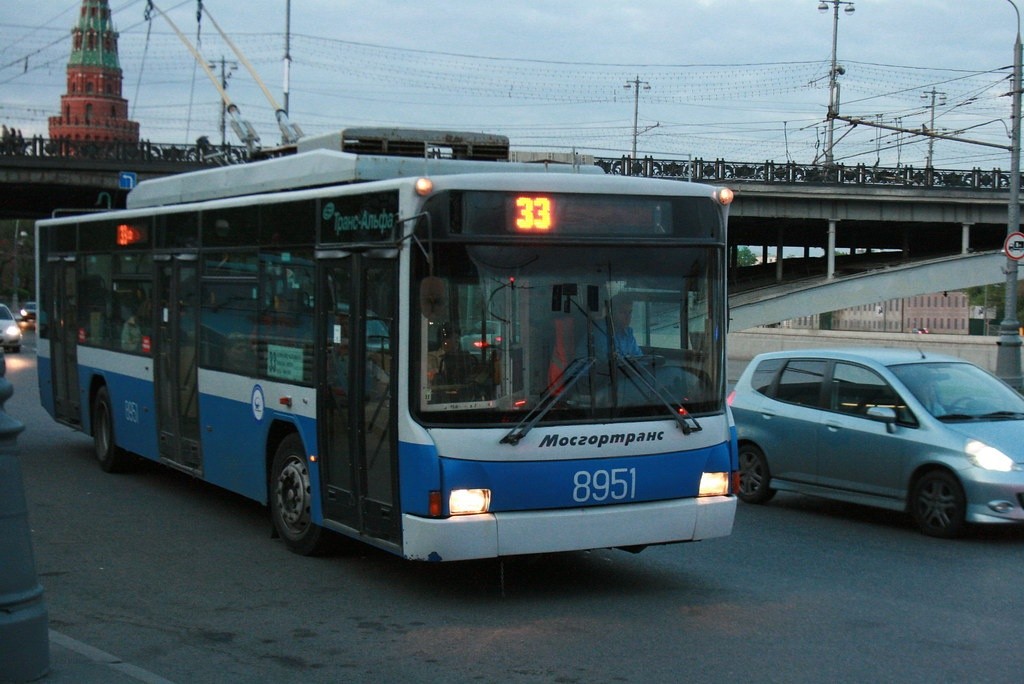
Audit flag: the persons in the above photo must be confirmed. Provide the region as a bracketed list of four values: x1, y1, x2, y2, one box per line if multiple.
[332, 315, 376, 403]
[574, 293, 646, 379]
[223, 332, 252, 370]
[427, 322, 483, 402]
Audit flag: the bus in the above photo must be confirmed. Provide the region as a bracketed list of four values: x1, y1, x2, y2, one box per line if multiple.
[34, 126, 737, 564]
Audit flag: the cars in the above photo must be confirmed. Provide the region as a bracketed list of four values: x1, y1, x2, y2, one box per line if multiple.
[728, 348, 1024, 538]
[0, 304, 22, 353]
[461, 318, 520, 361]
[23, 299, 36, 323]
[307, 299, 391, 351]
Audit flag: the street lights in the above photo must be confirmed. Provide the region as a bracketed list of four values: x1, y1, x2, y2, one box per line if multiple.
[206, 53, 240, 160]
[817, 0, 854, 179]
[622, 75, 650, 174]
[921, 85, 945, 184]
[12, 228, 28, 311]
